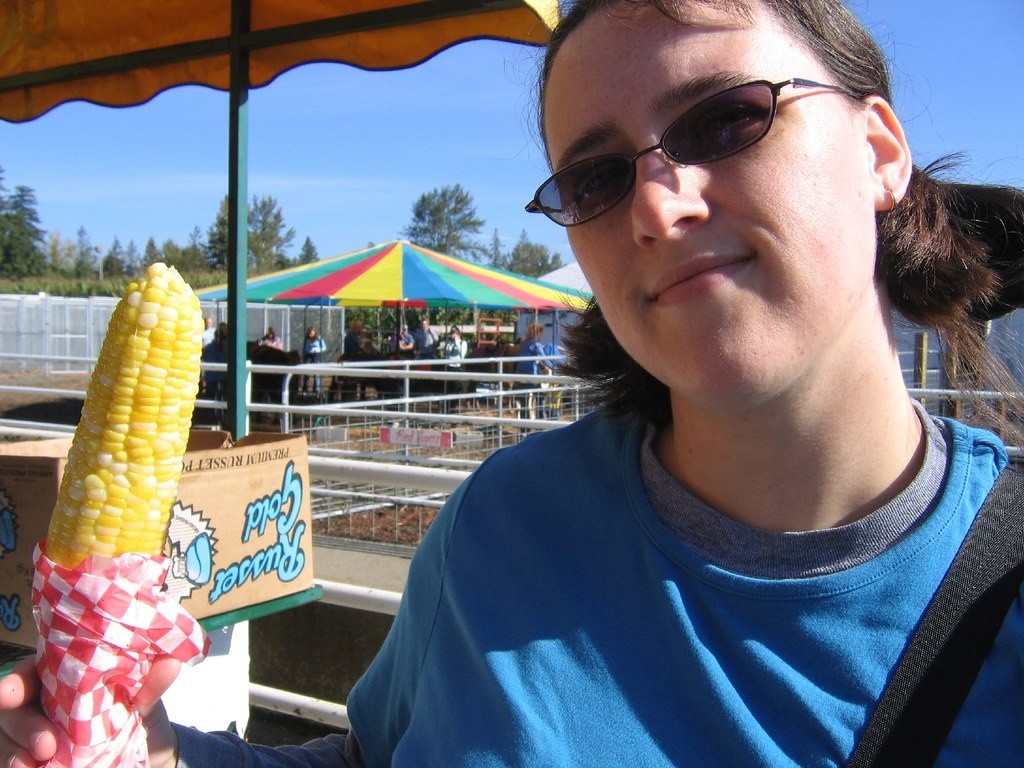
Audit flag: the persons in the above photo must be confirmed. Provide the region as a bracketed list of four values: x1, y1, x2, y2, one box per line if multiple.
[0, 0, 1024, 768]
[201, 319, 567, 435]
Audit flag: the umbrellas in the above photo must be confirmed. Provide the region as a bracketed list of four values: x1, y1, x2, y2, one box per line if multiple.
[195, 238, 596, 355]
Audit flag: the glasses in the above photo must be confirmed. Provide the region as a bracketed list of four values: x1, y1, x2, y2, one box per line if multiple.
[524, 77, 860, 228]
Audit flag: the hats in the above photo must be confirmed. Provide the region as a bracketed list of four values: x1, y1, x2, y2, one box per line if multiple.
[448, 326, 461, 335]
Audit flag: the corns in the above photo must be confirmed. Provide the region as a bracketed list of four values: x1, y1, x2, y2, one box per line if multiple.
[41, 263, 203, 570]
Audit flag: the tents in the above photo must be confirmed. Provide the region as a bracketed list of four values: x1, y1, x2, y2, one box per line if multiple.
[0, 0, 560, 443]
